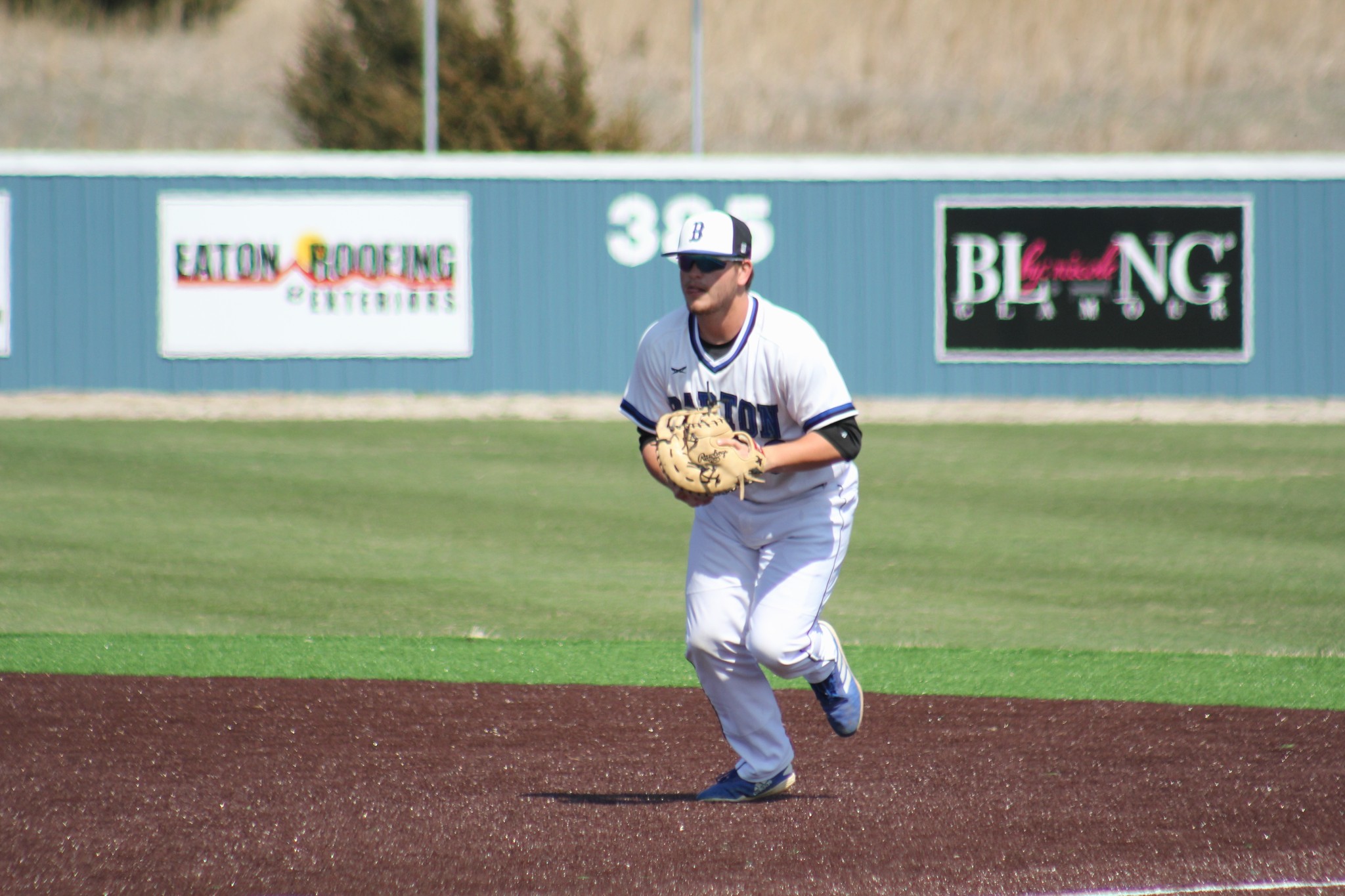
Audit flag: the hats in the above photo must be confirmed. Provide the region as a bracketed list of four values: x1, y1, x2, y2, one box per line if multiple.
[661, 209, 751, 262]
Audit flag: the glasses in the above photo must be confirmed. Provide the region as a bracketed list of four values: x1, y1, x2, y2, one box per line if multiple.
[676, 255, 741, 273]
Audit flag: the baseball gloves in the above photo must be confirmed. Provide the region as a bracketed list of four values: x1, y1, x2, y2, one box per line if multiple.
[654, 407, 767, 495]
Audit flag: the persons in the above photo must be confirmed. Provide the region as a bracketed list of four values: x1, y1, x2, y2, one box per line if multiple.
[621, 211, 864, 799]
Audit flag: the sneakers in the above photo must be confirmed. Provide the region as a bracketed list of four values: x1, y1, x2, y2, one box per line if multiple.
[810, 621, 864, 737]
[696, 763, 796, 802]
